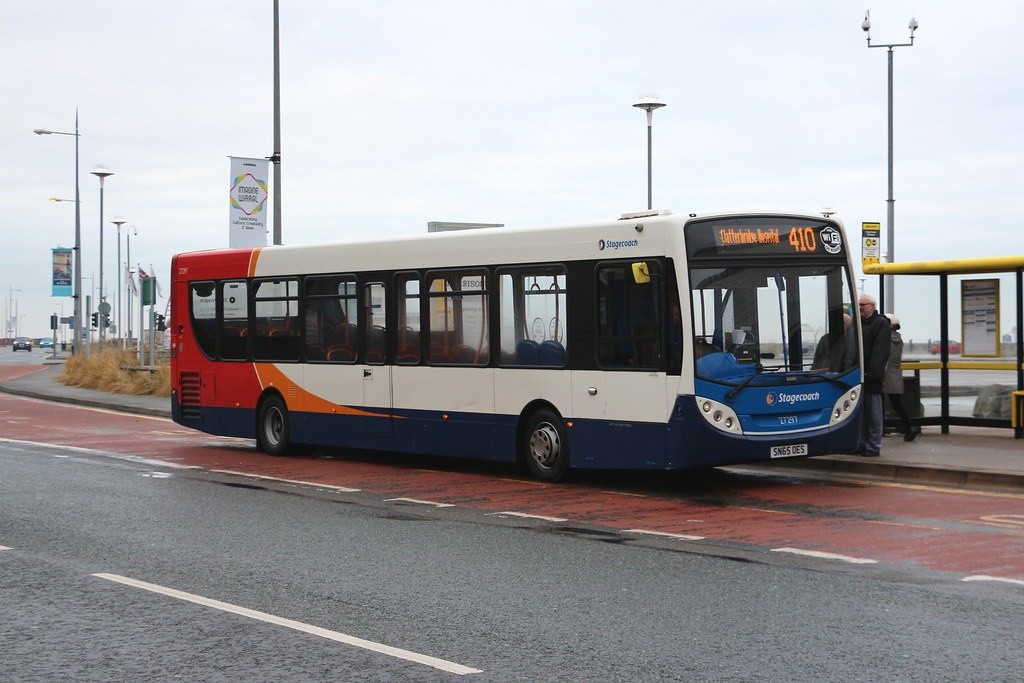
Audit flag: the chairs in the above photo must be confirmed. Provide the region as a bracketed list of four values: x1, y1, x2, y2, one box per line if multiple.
[516, 339, 565, 366]
[224, 323, 488, 364]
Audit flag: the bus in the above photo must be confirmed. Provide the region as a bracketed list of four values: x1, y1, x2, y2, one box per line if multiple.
[169, 209, 864, 483]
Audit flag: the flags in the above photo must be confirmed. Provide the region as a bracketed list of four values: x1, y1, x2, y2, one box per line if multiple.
[124, 264, 164, 297]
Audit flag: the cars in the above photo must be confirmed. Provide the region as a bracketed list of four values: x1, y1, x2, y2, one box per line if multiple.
[39, 337, 54, 348]
[12, 337, 34, 352]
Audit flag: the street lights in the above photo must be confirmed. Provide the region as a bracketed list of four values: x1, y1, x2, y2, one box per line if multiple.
[33, 106, 82, 355]
[89, 167, 115, 343]
[111, 217, 128, 339]
[862, 9, 919, 314]
[631, 96, 667, 211]
[123, 225, 138, 352]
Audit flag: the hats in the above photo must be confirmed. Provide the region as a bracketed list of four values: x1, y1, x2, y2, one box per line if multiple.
[884, 313, 900, 328]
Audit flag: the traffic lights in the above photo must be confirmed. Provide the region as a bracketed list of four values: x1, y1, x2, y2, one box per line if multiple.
[104, 313, 111, 328]
[91, 312, 98, 327]
[159, 315, 167, 331]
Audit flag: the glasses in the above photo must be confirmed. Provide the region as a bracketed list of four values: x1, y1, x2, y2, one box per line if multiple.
[860, 303, 871, 307]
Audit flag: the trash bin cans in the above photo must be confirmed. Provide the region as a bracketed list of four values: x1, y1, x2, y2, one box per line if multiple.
[883, 359, 921, 434]
[62, 341, 66, 351]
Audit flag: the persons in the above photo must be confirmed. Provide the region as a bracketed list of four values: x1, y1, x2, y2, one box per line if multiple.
[644, 304, 684, 368]
[810, 292, 918, 457]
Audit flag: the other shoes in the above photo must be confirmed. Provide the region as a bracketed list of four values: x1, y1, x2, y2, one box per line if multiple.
[854, 447, 880, 457]
[904, 427, 917, 441]
